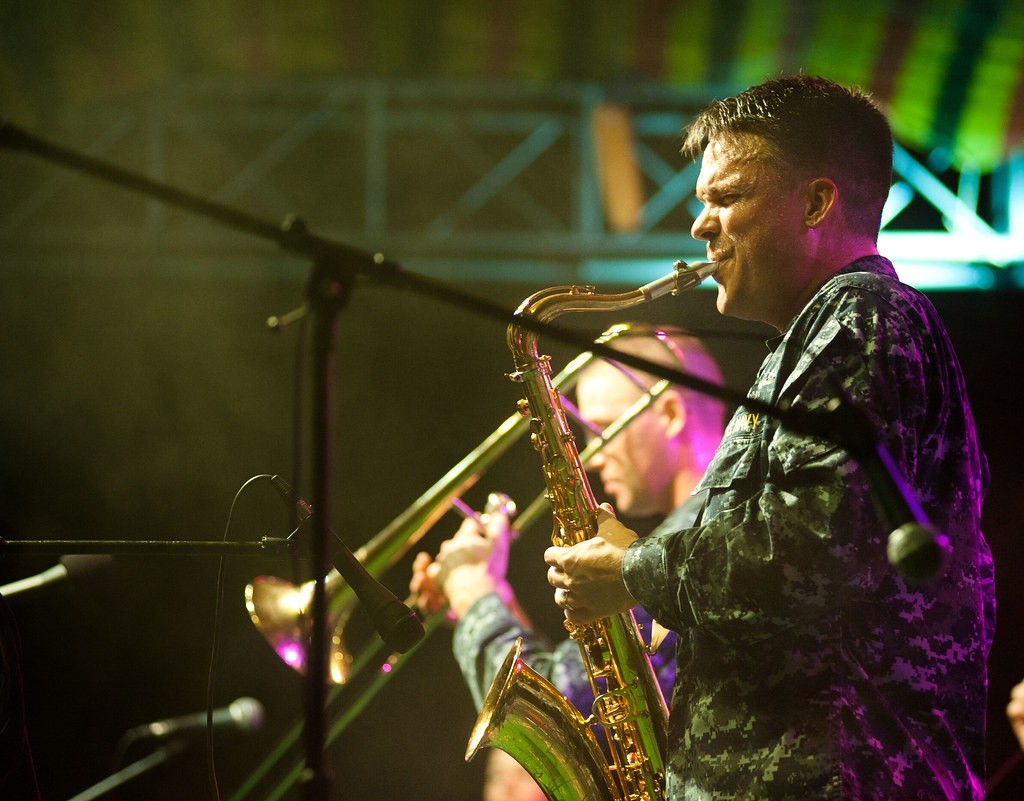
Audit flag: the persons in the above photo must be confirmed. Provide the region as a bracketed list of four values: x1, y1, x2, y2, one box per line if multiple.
[544, 77, 997, 801]
[410, 325, 725, 801]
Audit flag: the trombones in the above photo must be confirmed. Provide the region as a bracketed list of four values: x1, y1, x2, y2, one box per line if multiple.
[228, 321, 689, 801]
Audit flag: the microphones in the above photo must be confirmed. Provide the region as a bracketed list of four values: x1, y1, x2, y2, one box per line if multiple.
[143, 697, 265, 737]
[0, 552, 139, 613]
[828, 378, 946, 585]
[271, 475, 426, 653]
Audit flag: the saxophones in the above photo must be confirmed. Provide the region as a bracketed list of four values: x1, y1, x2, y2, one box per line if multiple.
[466, 257, 723, 801]
